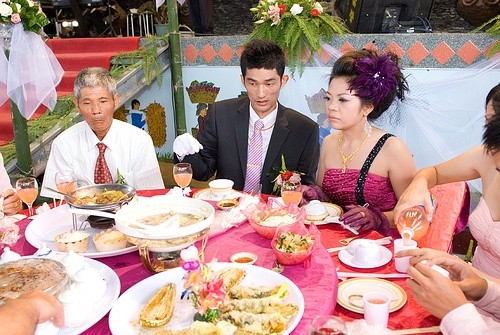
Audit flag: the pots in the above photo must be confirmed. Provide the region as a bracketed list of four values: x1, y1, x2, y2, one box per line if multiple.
[65, 183, 136, 222]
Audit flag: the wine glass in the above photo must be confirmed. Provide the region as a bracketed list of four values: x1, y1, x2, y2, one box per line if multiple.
[173, 163, 193, 196]
[15, 178, 39, 222]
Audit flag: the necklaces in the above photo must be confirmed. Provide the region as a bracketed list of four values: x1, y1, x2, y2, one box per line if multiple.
[249, 123, 275, 131]
[339, 126, 371, 173]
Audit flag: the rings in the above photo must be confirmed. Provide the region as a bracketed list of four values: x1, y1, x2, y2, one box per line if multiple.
[360, 212, 365, 217]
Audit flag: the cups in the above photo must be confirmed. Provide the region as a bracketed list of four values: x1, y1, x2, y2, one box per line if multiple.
[393, 238, 419, 273]
[351, 240, 383, 266]
[307, 315, 348, 335]
[281, 180, 302, 208]
[364, 292, 390, 329]
[55, 170, 77, 204]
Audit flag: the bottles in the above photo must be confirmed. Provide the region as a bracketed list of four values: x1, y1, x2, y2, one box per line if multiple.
[396, 192, 438, 240]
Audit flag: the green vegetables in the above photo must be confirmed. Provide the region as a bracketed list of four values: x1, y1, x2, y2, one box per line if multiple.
[275, 233, 308, 253]
[191, 290, 218, 323]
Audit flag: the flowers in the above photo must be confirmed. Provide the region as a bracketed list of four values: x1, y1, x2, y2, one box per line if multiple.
[0, 0, 51, 35]
[115, 170, 128, 186]
[264, 153, 307, 196]
[240, 0, 360, 81]
[348, 57, 399, 109]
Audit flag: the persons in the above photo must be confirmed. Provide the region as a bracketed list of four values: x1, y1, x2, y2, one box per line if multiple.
[0, 152, 22, 215]
[173, 38, 320, 197]
[394, 82, 500, 281]
[0, 290, 64, 335]
[40, 67, 166, 201]
[395, 116, 500, 335]
[299, 48, 417, 238]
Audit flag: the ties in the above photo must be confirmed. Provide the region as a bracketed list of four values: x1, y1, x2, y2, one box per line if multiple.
[244, 117, 265, 198]
[93, 143, 113, 185]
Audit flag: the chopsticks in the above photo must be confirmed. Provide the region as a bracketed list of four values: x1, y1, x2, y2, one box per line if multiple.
[335, 272, 413, 278]
[384, 326, 441, 335]
[327, 236, 392, 251]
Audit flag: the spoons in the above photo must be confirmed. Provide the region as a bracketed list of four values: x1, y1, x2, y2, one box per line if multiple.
[348, 294, 401, 309]
[324, 216, 359, 234]
[347, 239, 391, 254]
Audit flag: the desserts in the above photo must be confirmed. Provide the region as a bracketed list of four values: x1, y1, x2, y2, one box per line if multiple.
[56, 229, 95, 253]
[93, 228, 128, 253]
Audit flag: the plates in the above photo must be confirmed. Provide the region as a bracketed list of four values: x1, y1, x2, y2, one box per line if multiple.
[299, 202, 343, 226]
[108, 261, 304, 335]
[25, 204, 140, 259]
[216, 199, 238, 210]
[0, 253, 121, 335]
[192, 188, 243, 201]
[230, 252, 258, 265]
[338, 242, 393, 268]
[336, 279, 408, 314]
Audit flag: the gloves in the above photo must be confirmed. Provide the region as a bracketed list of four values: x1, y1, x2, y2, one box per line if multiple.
[173, 132, 203, 157]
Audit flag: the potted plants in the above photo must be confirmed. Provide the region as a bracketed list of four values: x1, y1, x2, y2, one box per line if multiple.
[137, 1, 194, 36]
[110, 33, 169, 89]
[0, 95, 80, 169]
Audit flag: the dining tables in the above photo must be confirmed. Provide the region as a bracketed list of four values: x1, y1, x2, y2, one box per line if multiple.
[0, 187, 470, 335]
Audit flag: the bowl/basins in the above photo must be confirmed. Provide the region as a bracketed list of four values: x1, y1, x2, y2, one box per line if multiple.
[240, 202, 304, 239]
[208, 179, 234, 197]
[113, 195, 214, 252]
[305, 200, 329, 220]
[0, 258, 69, 303]
[270, 220, 321, 265]
